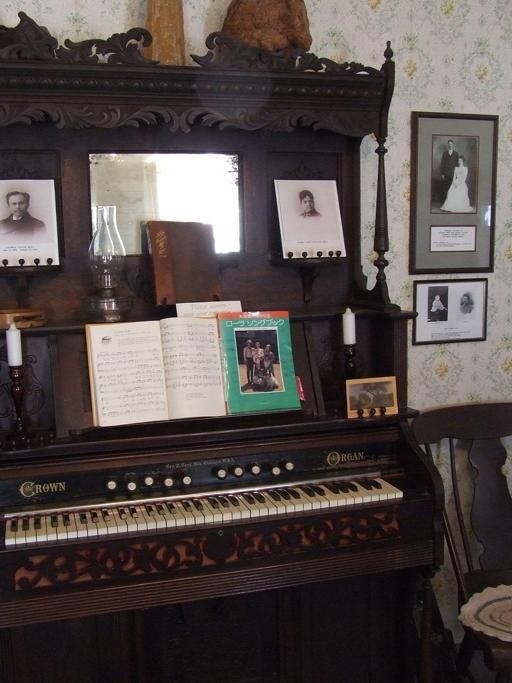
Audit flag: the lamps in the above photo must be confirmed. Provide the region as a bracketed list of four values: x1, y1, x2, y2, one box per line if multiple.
[82, 206, 126, 319]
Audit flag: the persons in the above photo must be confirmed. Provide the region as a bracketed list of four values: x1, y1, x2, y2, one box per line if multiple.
[1, 190, 48, 241]
[429, 293, 446, 312]
[295, 185, 323, 217]
[459, 293, 474, 315]
[243, 335, 281, 393]
[439, 138, 459, 202]
[439, 155, 474, 213]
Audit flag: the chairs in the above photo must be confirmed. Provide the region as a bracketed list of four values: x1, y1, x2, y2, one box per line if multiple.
[411, 402, 512, 682]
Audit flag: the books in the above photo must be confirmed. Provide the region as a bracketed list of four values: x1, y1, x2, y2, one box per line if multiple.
[84, 317, 227, 429]
[215, 308, 302, 418]
[143, 218, 225, 305]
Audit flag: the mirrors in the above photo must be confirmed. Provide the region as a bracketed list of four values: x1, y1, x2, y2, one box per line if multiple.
[89, 149, 243, 263]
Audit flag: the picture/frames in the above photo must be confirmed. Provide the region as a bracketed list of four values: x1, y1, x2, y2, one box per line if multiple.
[409, 110, 499, 274]
[414, 277, 490, 344]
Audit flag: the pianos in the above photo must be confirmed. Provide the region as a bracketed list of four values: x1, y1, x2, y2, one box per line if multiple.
[0, 407, 444, 683]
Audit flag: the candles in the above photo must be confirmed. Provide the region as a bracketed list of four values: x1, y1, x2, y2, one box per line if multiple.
[342, 306, 356, 344]
[5, 322, 22, 367]
